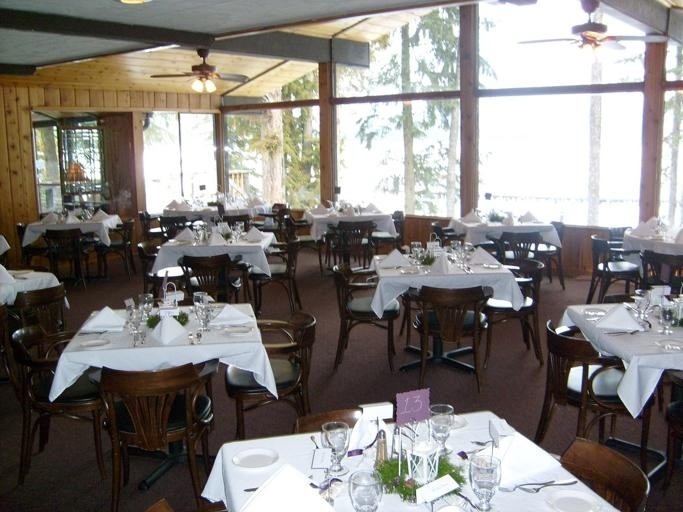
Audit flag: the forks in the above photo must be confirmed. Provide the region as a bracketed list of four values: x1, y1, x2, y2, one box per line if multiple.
[498, 477, 579, 496]
[76, 330, 108, 336]
[467, 433, 504, 454]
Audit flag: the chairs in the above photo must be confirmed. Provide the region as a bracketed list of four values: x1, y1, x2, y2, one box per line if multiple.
[13, 282, 68, 342]
[418, 283, 492, 393]
[10, 324, 108, 485]
[610, 227, 634, 260]
[586, 233, 643, 304]
[533, 320, 656, 474]
[559, 436, 651, 512]
[14, 201, 566, 291]
[145, 498, 174, 512]
[226, 312, 316, 440]
[100, 358, 219, 511]
[176, 253, 235, 306]
[294, 401, 397, 433]
[666, 404, 682, 474]
[246, 237, 304, 313]
[330, 264, 403, 377]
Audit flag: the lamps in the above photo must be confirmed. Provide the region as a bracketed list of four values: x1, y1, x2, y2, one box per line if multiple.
[115, 0, 152, 4]
[191, 77, 216, 93]
[576, 40, 601, 51]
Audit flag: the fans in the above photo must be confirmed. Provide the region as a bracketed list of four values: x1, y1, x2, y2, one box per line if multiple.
[516, 0, 669, 50]
[150, 48, 248, 83]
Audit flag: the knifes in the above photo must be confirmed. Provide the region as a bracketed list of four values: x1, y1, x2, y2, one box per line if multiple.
[242, 473, 312, 492]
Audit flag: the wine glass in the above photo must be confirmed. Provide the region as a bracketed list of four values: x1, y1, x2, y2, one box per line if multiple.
[348, 468, 385, 511]
[465, 452, 501, 511]
[190, 192, 246, 210]
[325, 198, 363, 215]
[128, 293, 153, 347]
[427, 403, 454, 457]
[319, 420, 349, 475]
[408, 239, 475, 272]
[192, 291, 211, 332]
[188, 216, 245, 244]
[632, 287, 682, 335]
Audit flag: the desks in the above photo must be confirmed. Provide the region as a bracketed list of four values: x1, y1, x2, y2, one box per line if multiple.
[369, 245, 525, 375]
[1, 236, 10, 256]
[1, 270, 62, 308]
[622, 226, 681, 297]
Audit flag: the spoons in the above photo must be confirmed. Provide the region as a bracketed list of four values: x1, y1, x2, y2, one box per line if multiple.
[605, 329, 637, 338]
[395, 422, 418, 441]
[309, 434, 318, 449]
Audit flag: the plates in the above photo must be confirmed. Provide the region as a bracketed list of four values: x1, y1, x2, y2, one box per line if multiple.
[585, 307, 607, 317]
[481, 263, 500, 270]
[232, 446, 278, 470]
[223, 326, 253, 336]
[400, 267, 429, 275]
[656, 338, 682, 350]
[450, 414, 467, 430]
[13, 269, 32, 277]
[80, 338, 110, 348]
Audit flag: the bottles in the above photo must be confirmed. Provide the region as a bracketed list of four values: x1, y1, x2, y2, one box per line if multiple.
[376, 429, 388, 462]
[390, 427, 400, 461]
